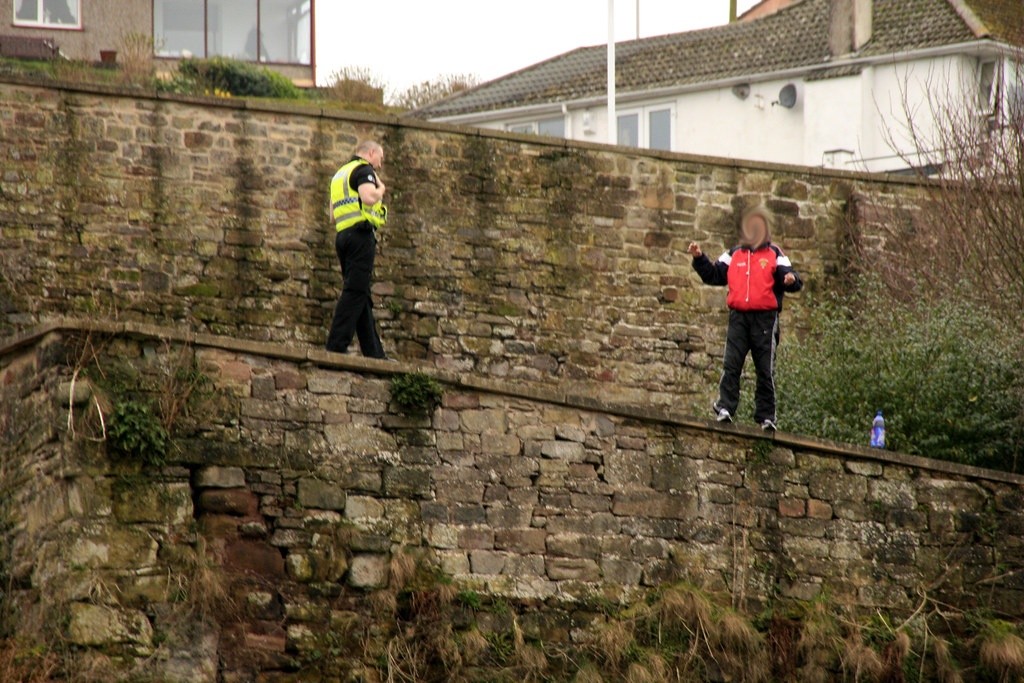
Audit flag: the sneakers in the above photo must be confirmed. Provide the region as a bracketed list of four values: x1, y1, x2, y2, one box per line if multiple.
[716, 409, 733, 423]
[761, 419, 777, 433]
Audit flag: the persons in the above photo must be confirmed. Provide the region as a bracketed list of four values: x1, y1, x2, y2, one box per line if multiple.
[687, 206, 804, 432]
[243, 25, 271, 63]
[325, 140, 389, 362]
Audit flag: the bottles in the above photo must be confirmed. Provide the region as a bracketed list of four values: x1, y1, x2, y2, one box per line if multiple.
[870, 411, 886, 448]
